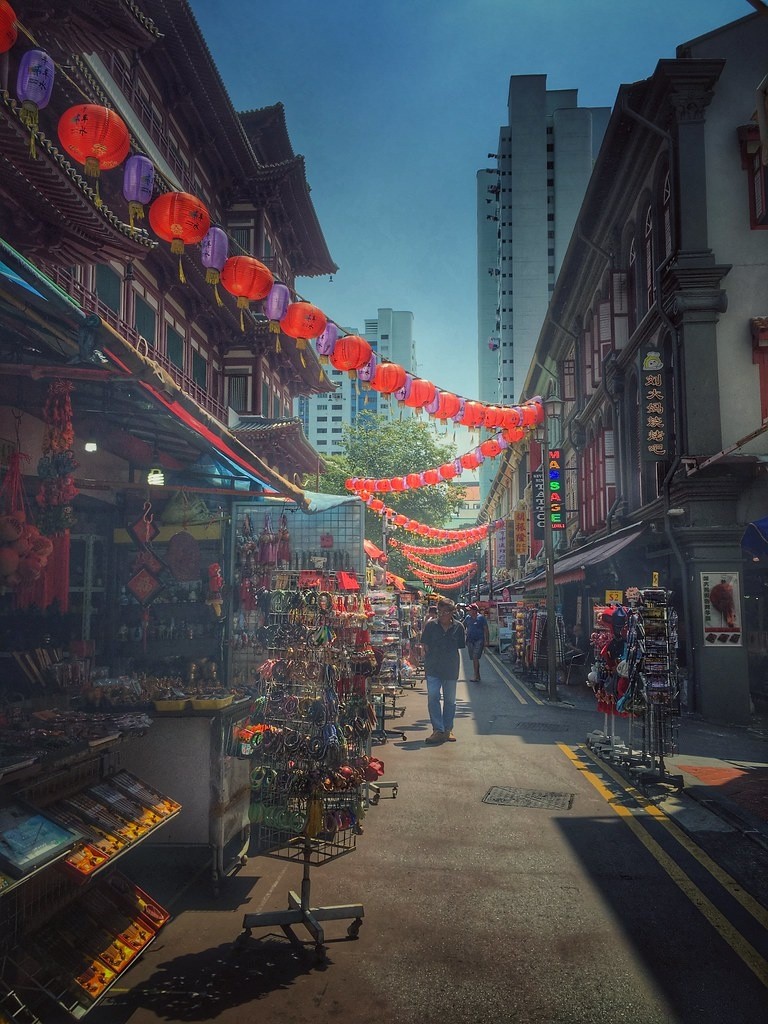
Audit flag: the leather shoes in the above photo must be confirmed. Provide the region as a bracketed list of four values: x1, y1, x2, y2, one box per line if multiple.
[426, 730, 457, 743]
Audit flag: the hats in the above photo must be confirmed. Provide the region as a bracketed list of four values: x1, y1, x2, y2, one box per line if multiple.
[465, 604, 479, 610]
[600, 602, 632, 699]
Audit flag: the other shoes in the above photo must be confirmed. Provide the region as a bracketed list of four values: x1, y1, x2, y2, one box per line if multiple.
[470, 675, 480, 681]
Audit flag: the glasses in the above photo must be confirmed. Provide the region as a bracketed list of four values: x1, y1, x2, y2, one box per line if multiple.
[436, 610, 451, 616]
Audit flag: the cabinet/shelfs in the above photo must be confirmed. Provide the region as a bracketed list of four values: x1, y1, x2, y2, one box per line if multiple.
[586, 587, 684, 794]
[0, 562, 423, 1024]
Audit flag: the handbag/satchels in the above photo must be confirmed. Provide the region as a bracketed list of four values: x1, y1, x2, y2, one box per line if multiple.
[616, 608, 648, 716]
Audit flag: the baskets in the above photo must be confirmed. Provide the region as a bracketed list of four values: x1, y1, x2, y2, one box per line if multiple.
[153, 694, 235, 711]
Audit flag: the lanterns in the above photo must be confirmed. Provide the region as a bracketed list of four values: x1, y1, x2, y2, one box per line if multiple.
[0, 0, 545, 589]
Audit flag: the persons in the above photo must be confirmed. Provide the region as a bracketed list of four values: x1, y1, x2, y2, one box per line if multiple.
[421, 598, 489, 745]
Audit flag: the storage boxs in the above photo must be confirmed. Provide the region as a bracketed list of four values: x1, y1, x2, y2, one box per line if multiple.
[152, 695, 195, 711]
[191, 694, 235, 710]
